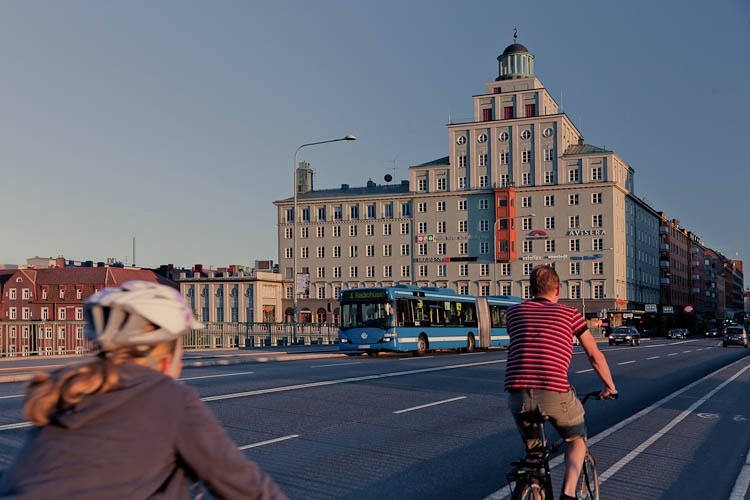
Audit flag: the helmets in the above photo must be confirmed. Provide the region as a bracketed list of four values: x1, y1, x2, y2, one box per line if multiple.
[79, 279, 206, 348]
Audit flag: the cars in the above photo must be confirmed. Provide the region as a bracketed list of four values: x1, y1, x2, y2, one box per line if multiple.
[667, 329, 686, 340]
[609, 327, 641, 346]
[722, 325, 748, 348]
[705, 328, 720, 337]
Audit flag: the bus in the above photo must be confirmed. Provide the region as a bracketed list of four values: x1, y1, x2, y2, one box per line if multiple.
[338, 285, 523, 357]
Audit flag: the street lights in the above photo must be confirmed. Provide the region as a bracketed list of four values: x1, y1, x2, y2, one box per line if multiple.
[494, 214, 537, 296]
[582, 248, 614, 319]
[293, 134, 357, 323]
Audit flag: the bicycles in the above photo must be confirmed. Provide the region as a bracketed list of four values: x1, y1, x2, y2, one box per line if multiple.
[506, 390, 621, 500]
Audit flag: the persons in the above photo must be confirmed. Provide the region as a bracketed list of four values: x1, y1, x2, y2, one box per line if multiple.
[0, 280, 289, 500]
[505, 265, 618, 500]
